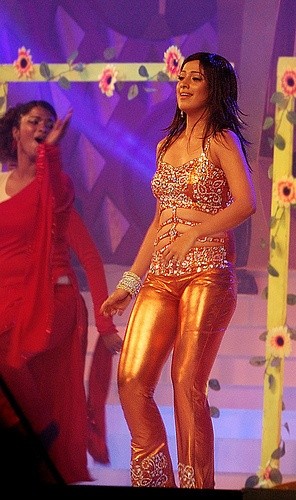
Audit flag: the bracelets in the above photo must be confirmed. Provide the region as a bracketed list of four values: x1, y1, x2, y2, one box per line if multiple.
[116, 271, 144, 299]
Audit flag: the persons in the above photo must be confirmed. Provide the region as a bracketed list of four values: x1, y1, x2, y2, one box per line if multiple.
[97, 50, 257, 488]
[0, 99, 123, 485]
